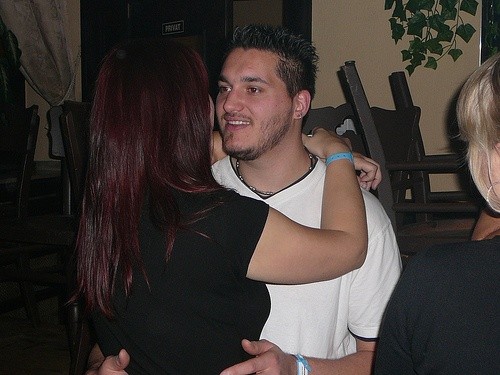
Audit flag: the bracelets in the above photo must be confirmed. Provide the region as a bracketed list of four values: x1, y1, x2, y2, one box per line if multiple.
[292, 353, 312, 375]
[325, 151, 354, 167]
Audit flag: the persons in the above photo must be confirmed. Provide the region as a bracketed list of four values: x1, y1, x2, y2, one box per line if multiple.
[62, 32, 383, 375]
[81, 21, 405, 375]
[372, 51, 500, 375]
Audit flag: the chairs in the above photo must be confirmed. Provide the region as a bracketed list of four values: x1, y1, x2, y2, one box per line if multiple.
[0, 101, 94, 375]
[302, 60, 485, 271]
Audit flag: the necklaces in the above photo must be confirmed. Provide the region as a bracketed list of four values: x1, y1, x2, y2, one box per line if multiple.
[235, 147, 312, 196]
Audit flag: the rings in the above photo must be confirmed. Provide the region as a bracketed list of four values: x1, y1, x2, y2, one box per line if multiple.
[311, 126, 319, 136]
